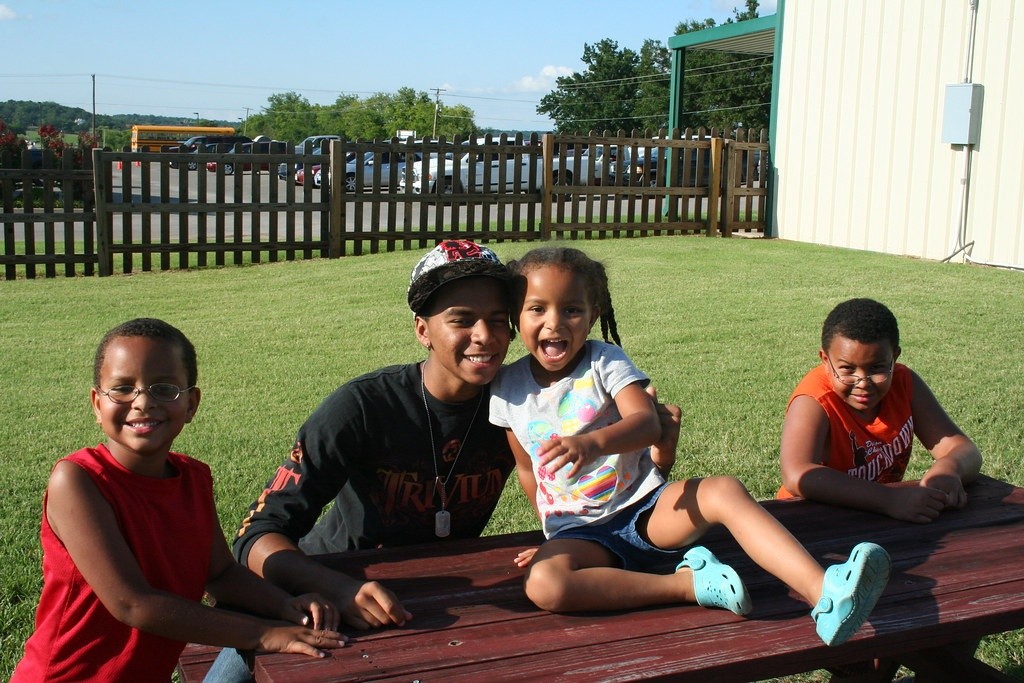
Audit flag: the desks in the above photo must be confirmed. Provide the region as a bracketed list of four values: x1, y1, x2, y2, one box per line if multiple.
[208, 470, 1024, 683]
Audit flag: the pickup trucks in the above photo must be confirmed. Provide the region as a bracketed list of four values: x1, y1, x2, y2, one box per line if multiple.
[398, 135, 594, 204]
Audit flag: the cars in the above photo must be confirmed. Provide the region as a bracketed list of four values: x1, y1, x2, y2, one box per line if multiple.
[279, 135, 459, 193]
[604, 140, 757, 198]
[580, 144, 654, 183]
[207, 141, 292, 176]
[188, 142, 234, 171]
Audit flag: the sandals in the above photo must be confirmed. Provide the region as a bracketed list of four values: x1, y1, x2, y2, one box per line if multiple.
[810, 541, 888, 644]
[676, 547, 753, 614]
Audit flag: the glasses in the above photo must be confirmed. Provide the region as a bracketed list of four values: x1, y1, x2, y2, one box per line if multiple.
[826, 351, 895, 385]
[95, 383, 195, 404]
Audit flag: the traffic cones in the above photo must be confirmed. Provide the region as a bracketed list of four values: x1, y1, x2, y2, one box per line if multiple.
[117, 161, 123, 170]
[135, 161, 140, 167]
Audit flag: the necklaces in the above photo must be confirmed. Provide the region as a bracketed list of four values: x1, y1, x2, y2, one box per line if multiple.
[421, 359, 484, 537]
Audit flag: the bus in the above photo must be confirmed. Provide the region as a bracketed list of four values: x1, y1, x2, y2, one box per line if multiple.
[131, 124, 235, 153]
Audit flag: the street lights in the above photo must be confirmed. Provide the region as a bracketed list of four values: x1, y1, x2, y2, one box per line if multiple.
[193, 112, 199, 126]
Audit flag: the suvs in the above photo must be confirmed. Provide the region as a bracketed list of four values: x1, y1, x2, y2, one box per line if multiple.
[168, 136, 254, 170]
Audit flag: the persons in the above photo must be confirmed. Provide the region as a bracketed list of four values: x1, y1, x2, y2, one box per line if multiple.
[231, 239, 682, 631]
[488, 247, 892, 649]
[776, 297, 983, 526]
[8, 317, 349, 683]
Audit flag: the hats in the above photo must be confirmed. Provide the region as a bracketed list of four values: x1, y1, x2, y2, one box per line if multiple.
[408, 239, 527, 330]
